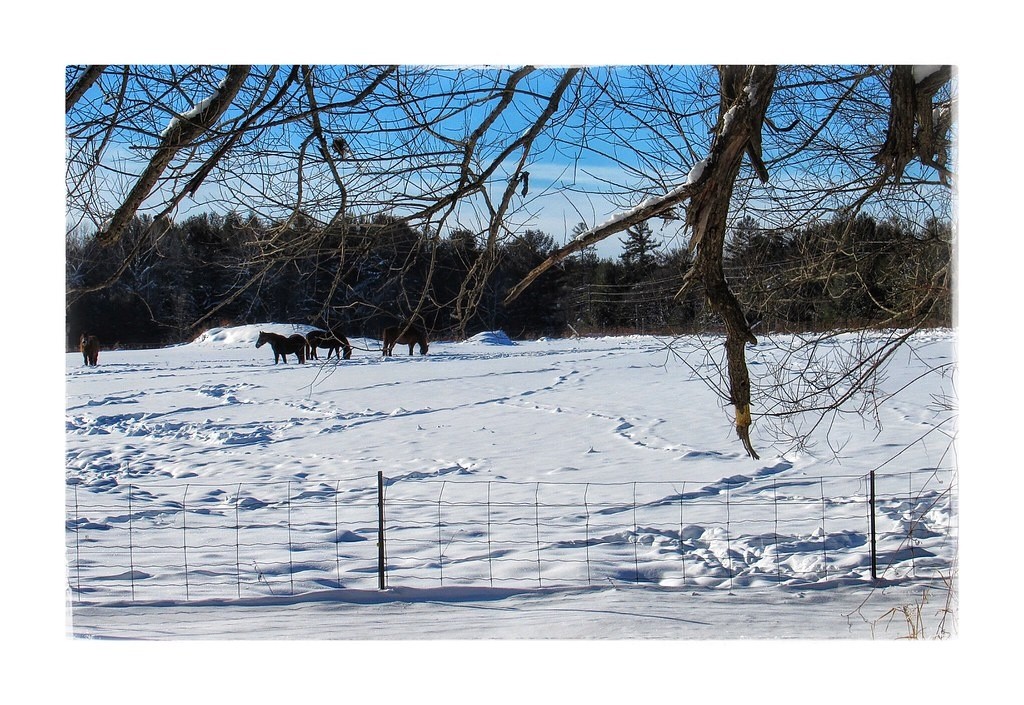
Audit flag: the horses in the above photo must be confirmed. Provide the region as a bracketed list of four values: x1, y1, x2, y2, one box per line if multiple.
[382, 324, 429, 356]
[306, 330, 352, 360]
[79, 328, 100, 366]
[256, 331, 310, 364]
